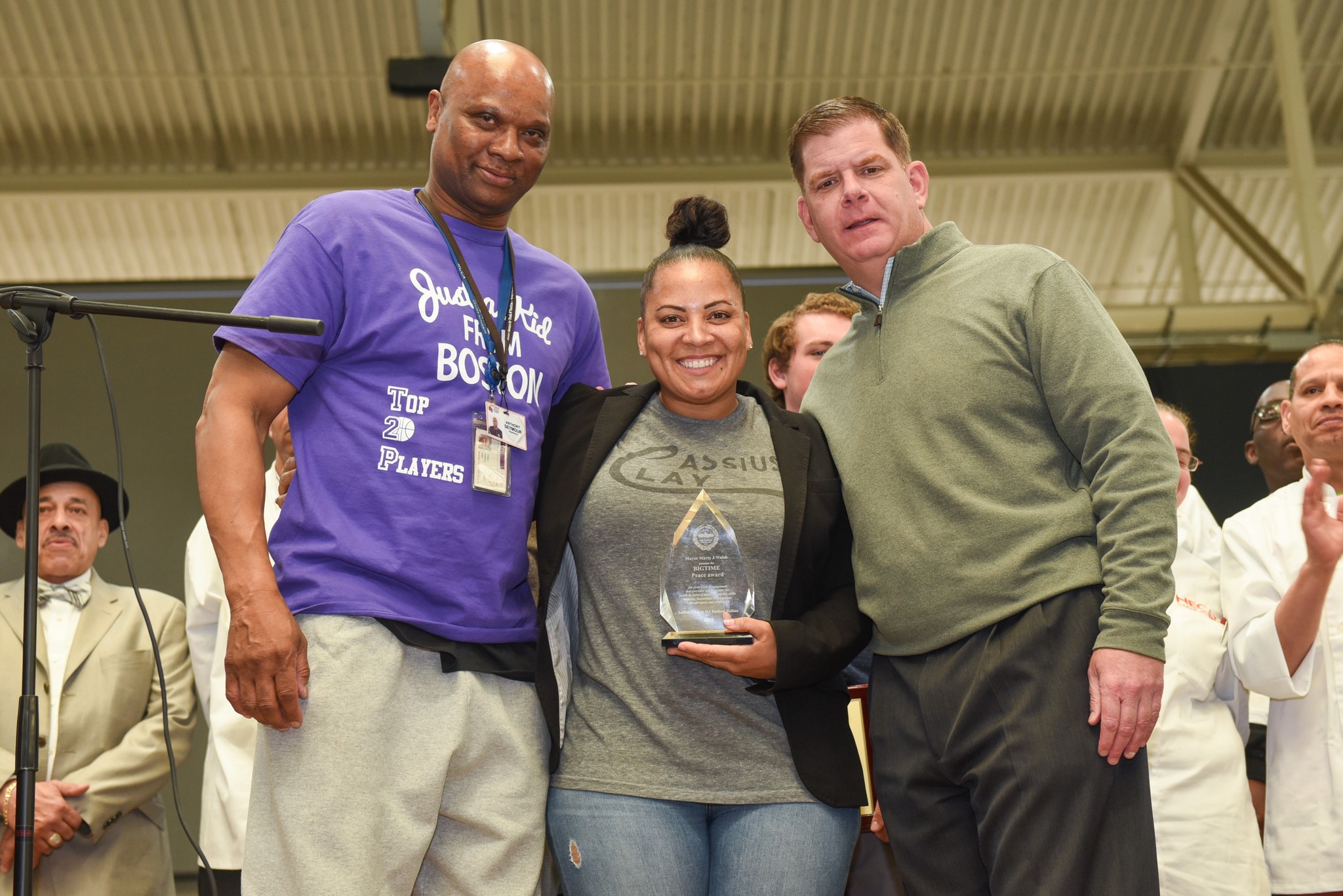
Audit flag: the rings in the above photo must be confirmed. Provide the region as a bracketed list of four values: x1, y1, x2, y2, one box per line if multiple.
[47, 833, 61, 847]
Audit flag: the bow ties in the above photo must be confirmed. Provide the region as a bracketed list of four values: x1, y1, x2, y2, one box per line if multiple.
[36, 583, 92, 609]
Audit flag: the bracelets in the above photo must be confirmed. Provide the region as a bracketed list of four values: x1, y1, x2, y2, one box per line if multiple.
[3, 781, 17, 826]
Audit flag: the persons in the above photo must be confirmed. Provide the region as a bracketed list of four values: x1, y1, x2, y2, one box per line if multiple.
[0, 443, 197, 896]
[276, 196, 872, 896]
[763, 292, 906, 896]
[184, 404, 293, 896]
[788, 95, 1181, 896]
[1145, 339, 1343, 896]
[195, 38, 611, 896]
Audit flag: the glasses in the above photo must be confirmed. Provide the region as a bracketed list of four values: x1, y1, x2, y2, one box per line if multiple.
[1249, 400, 1282, 438]
[1176, 449, 1203, 472]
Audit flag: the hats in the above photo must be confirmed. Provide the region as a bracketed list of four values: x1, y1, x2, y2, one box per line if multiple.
[0, 443, 129, 539]
